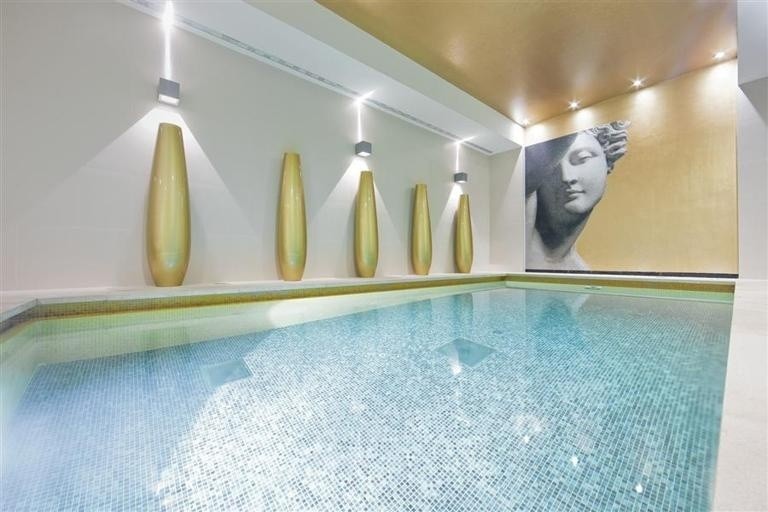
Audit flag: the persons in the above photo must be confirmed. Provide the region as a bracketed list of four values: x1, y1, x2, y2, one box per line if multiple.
[525, 122, 628, 270]
